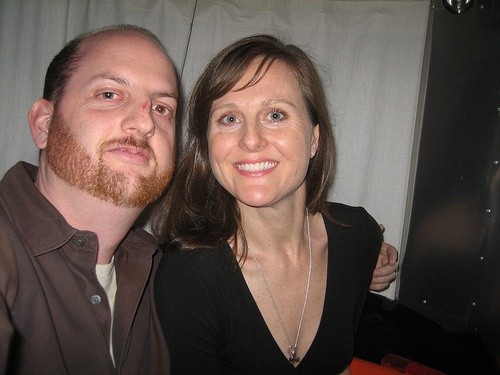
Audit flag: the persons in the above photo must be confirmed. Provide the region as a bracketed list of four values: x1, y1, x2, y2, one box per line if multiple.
[151, 34, 383, 375]
[0, 24, 399, 375]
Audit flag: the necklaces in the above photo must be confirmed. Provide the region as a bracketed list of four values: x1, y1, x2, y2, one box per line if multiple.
[241, 205, 312, 367]
